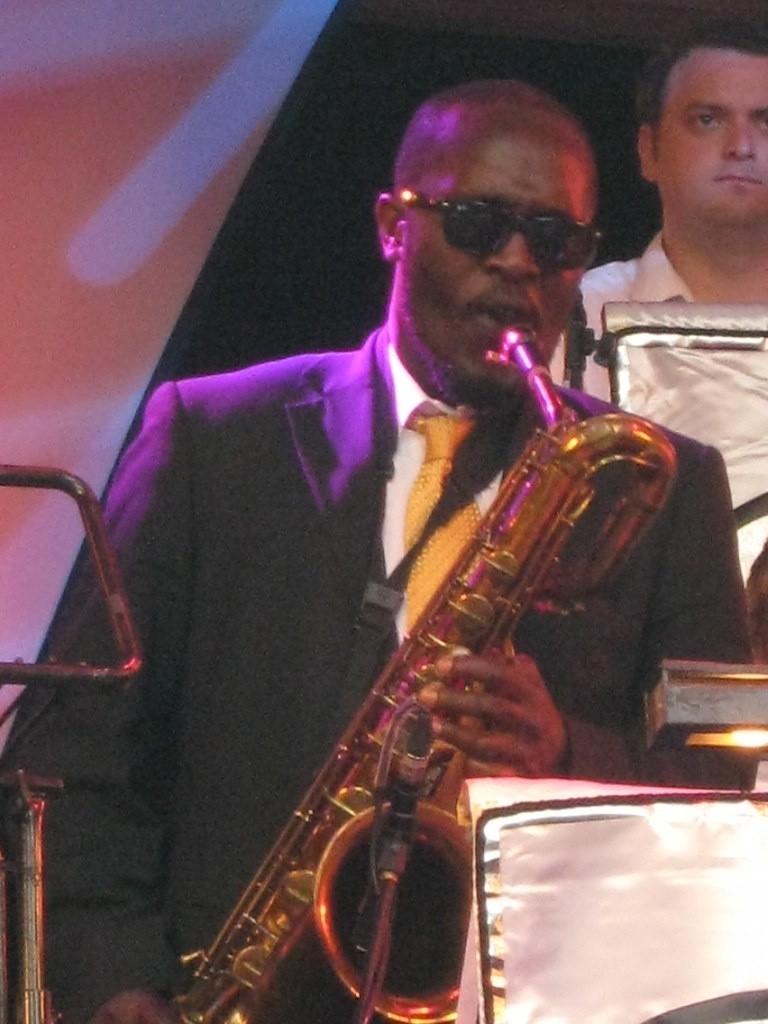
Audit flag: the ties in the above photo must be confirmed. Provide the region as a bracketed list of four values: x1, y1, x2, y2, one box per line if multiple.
[403, 411, 486, 634]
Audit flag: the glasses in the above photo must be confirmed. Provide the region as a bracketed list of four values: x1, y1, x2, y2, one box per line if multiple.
[398, 189, 600, 266]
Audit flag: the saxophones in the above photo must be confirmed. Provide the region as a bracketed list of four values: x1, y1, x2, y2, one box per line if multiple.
[168, 318, 681, 1022]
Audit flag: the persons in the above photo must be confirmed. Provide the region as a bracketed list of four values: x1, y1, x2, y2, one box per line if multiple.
[0, 79, 764, 1024]
[550, 18, 768, 551]
